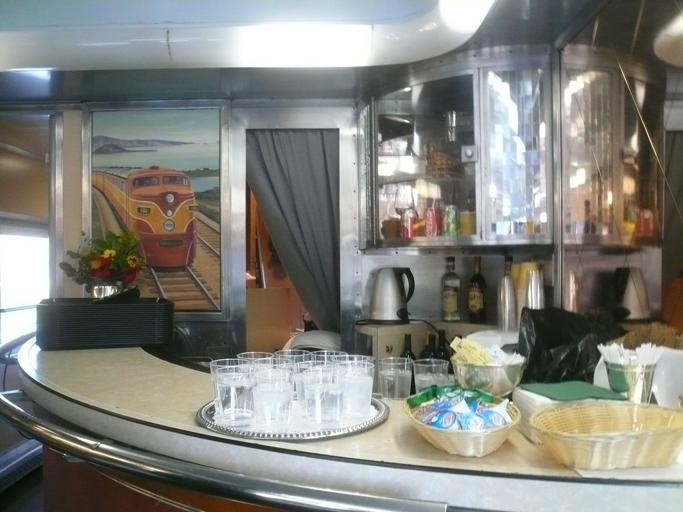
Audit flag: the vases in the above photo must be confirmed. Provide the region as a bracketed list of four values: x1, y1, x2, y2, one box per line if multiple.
[92, 286, 117, 299]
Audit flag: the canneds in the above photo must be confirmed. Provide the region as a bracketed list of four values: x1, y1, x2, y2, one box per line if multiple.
[423, 208, 440, 237]
[441, 204, 461, 238]
[402, 209, 420, 237]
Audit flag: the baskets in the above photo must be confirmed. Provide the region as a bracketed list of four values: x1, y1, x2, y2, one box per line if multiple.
[404, 393, 521, 458]
[530, 402, 683, 471]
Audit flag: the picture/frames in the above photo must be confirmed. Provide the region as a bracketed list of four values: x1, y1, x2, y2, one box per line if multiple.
[81, 99, 232, 322]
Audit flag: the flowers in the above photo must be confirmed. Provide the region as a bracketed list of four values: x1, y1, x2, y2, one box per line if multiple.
[59, 224, 146, 292]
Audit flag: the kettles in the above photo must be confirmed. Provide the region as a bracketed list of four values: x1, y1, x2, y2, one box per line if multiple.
[367, 266, 416, 325]
[609, 268, 652, 326]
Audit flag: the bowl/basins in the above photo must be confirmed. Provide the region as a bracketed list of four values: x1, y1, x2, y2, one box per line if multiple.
[450, 356, 527, 397]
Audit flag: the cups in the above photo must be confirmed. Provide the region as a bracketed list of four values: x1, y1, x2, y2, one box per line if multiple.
[603, 358, 657, 408]
[209, 349, 450, 434]
[460, 212, 476, 235]
[497, 261, 545, 332]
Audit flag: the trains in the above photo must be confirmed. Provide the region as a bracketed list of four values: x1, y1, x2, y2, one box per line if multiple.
[90, 165, 196, 268]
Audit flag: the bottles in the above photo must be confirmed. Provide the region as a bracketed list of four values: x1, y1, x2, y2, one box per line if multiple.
[467, 256, 487, 323]
[503, 256, 513, 277]
[400, 330, 461, 395]
[440, 256, 461, 323]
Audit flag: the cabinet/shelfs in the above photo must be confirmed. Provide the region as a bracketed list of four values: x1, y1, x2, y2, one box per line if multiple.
[358, 43, 664, 256]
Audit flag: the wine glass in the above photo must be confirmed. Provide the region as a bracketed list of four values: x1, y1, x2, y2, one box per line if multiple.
[394, 185, 414, 240]
